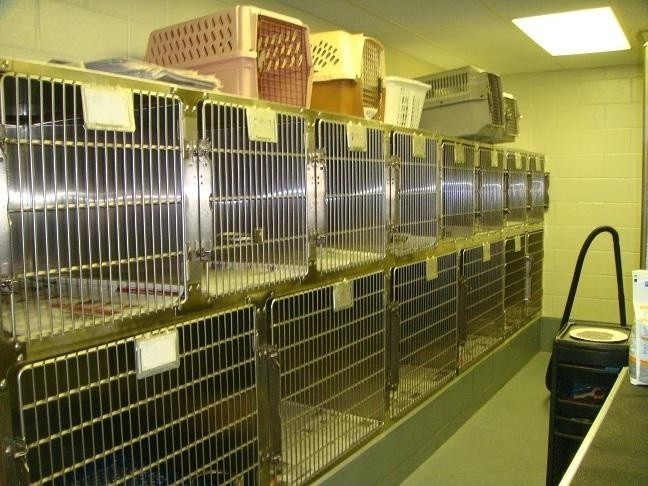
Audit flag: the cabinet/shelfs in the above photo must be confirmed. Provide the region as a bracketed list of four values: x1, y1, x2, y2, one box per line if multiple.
[545, 318, 633, 486]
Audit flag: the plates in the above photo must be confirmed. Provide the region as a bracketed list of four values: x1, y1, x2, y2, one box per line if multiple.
[568, 327, 629, 344]
